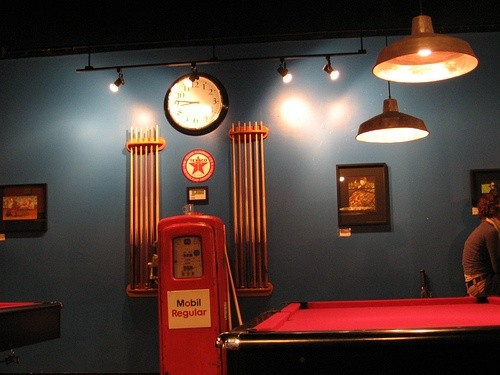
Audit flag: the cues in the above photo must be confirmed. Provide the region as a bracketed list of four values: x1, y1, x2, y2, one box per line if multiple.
[130, 124, 159, 288]
[222, 223, 242, 331]
[232, 120, 268, 288]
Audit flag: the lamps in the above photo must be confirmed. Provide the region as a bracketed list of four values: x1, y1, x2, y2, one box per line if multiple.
[189, 63, 199, 82]
[324, 56, 333, 74]
[356, 28, 430, 144]
[114, 67, 124, 87]
[372, 0, 480, 84]
[277, 57, 289, 77]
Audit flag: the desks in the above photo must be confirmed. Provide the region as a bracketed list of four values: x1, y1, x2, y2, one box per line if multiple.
[216, 294, 500, 375]
[0, 301, 63, 352]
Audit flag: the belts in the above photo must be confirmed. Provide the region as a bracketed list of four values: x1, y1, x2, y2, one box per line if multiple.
[466, 275, 489, 288]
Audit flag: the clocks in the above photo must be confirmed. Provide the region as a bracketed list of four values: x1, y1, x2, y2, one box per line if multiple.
[164, 72, 229, 136]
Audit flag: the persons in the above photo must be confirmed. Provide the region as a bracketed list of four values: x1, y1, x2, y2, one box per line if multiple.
[463, 189, 500, 298]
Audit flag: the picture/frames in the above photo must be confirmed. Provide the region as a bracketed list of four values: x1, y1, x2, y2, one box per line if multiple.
[0, 183, 48, 232]
[336, 163, 391, 226]
